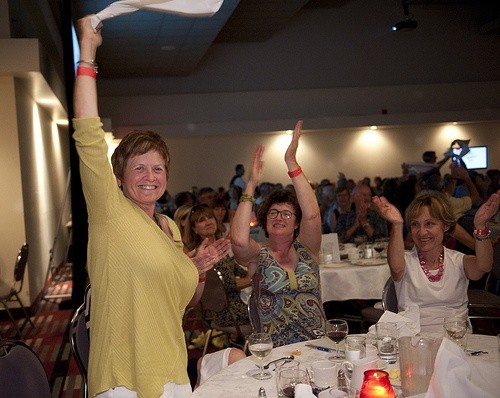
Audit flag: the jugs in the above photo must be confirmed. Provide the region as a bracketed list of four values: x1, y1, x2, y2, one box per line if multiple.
[342, 356, 378, 394]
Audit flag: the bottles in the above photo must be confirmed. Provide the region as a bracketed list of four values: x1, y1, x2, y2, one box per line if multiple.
[258, 387, 267, 398]
[337, 368, 350, 393]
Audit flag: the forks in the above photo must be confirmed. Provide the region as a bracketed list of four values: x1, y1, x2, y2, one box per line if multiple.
[256, 357, 289, 369]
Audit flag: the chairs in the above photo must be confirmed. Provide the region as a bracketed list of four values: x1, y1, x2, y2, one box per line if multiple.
[0, 214, 500, 398]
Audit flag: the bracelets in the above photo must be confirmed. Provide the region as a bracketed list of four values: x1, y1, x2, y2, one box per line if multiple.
[474, 225, 489, 236]
[76, 60, 98, 79]
[362, 222, 370, 228]
[473, 230, 492, 241]
[199, 277, 206, 283]
[240, 193, 256, 203]
[288, 168, 303, 177]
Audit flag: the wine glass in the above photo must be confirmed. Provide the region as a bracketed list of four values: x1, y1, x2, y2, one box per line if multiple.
[248, 333, 273, 380]
[326, 318, 348, 362]
[374, 240, 385, 259]
[442, 317, 467, 349]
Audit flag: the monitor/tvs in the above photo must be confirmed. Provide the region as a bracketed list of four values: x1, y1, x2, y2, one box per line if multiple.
[452, 145, 488, 170]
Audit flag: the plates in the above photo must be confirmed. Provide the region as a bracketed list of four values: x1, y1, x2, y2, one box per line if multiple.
[351, 259, 387, 265]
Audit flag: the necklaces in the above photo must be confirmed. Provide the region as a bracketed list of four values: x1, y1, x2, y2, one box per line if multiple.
[153, 214, 163, 230]
[417, 248, 444, 281]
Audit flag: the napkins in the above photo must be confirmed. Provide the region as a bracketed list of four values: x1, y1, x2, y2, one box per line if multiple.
[369, 304, 421, 337]
[427, 338, 500, 398]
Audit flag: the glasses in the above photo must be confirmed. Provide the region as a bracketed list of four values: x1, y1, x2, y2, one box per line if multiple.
[266, 209, 297, 220]
[189, 203, 210, 221]
[208, 194, 216, 199]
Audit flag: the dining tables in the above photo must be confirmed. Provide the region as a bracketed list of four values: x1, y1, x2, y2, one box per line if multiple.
[190, 328, 500, 398]
[336, 238, 400, 258]
[318, 251, 410, 330]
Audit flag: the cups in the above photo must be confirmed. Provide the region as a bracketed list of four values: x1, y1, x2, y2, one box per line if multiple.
[279, 370, 308, 398]
[345, 336, 367, 359]
[318, 390, 347, 398]
[324, 255, 332, 264]
[348, 253, 362, 264]
[399, 336, 442, 398]
[274, 359, 300, 398]
[311, 361, 337, 389]
[375, 321, 399, 364]
[345, 349, 360, 362]
[364, 243, 372, 259]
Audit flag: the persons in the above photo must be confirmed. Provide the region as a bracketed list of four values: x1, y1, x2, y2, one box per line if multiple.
[157, 119, 500, 391]
[71, 15, 198, 398]
